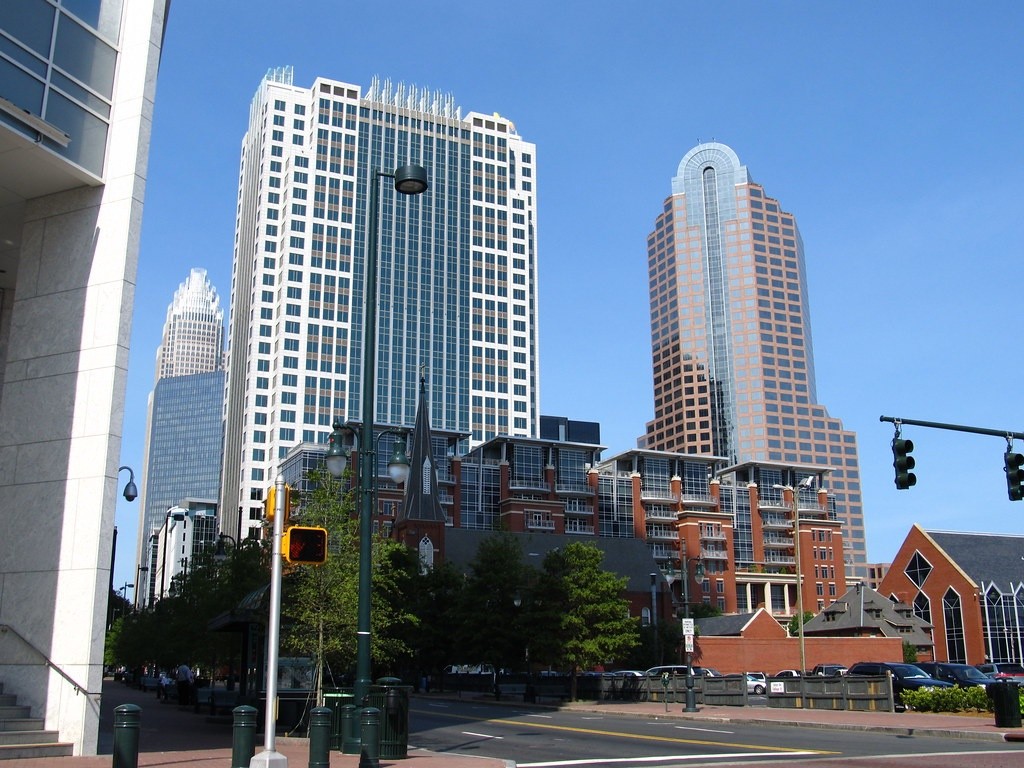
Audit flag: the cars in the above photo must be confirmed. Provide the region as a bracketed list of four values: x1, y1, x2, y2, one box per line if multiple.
[443, 660, 1024, 713]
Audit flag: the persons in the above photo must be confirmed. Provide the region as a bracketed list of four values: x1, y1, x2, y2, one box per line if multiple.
[294, 670, 305, 688]
[161, 659, 194, 711]
[203, 666, 228, 686]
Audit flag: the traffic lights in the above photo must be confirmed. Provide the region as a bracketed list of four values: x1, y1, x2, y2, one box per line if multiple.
[1004, 452, 1024, 501]
[282, 524, 328, 567]
[891, 437, 916, 490]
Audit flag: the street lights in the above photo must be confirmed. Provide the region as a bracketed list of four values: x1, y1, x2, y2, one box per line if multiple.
[772, 482, 809, 676]
[665, 535, 707, 712]
[324, 420, 410, 754]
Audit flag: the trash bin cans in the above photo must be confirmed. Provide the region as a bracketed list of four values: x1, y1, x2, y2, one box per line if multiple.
[320, 686, 355, 750]
[987, 677, 1022, 728]
[364, 677, 414, 760]
